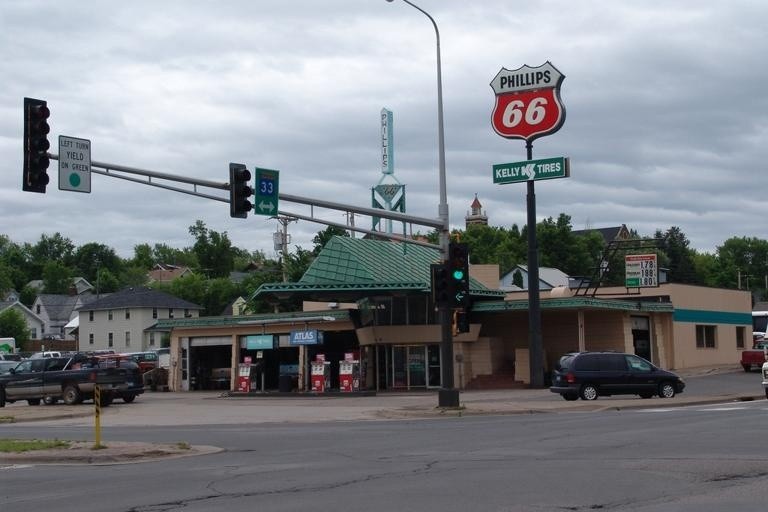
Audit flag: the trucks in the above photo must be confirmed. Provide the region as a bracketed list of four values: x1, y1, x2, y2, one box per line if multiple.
[740, 341, 768, 371]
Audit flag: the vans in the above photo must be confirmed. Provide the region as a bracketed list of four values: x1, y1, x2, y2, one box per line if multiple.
[551, 351, 687, 401]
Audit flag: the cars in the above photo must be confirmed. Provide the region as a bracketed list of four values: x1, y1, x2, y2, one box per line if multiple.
[761, 360, 768, 398]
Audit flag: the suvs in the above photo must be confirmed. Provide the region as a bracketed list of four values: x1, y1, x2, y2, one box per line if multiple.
[0, 350, 170, 409]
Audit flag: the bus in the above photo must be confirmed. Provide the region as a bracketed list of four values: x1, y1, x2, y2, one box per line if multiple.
[753, 310, 768, 345]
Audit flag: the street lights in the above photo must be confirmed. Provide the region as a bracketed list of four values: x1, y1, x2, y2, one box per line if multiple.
[383, 0, 461, 406]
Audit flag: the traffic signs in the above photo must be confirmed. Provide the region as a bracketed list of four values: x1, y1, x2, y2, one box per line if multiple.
[57, 132, 94, 195]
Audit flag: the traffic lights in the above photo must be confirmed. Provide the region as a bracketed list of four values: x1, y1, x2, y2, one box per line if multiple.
[449, 264, 471, 306]
[431, 263, 450, 306]
[24, 96, 51, 192]
[229, 162, 252, 218]
